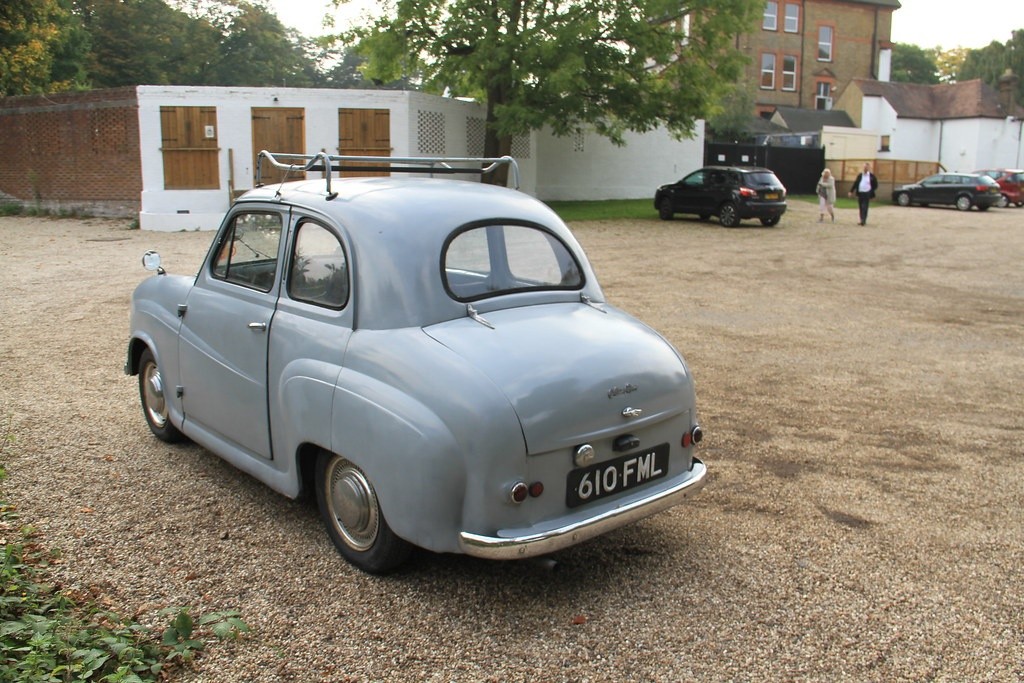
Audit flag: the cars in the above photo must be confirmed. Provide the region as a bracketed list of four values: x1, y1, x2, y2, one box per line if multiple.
[891, 173, 1002, 211]
[122, 149, 708, 575]
[969, 167, 1024, 209]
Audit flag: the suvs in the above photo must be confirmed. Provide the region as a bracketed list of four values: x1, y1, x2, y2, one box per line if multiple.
[654, 165, 787, 229]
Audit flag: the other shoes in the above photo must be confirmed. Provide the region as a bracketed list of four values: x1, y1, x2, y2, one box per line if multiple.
[831, 219, 834, 224]
[858, 221, 866, 226]
[817, 220, 823, 224]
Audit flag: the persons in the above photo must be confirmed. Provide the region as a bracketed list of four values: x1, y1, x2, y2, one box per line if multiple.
[848, 162, 878, 227]
[816, 169, 835, 223]
